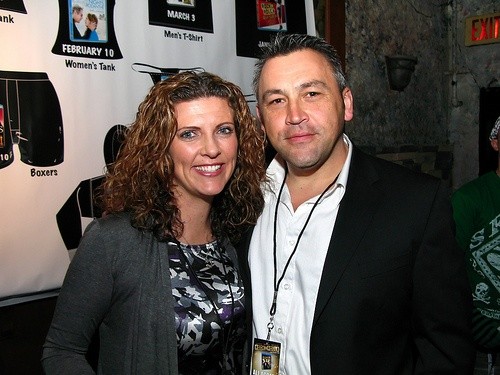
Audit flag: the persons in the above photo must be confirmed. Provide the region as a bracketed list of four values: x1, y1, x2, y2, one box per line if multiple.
[42, 70, 268, 375]
[233, 34, 500, 375]
[72, 7, 99, 39]
